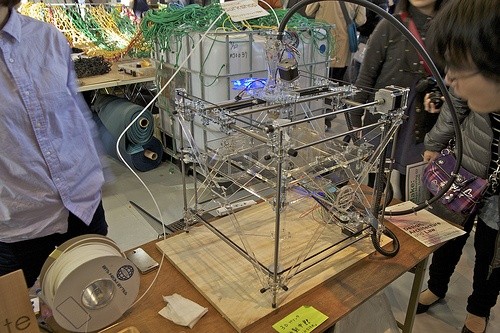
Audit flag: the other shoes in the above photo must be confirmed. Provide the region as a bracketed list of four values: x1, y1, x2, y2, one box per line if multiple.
[462, 313, 489, 333]
[416, 288, 442, 314]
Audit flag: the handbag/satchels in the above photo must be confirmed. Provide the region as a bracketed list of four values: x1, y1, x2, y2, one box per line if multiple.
[421, 147, 492, 217]
[348, 22, 359, 53]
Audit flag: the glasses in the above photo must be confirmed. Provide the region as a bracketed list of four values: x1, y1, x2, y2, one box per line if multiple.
[443, 63, 482, 84]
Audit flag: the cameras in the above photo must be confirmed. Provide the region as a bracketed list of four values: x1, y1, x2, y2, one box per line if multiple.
[415, 76, 444, 110]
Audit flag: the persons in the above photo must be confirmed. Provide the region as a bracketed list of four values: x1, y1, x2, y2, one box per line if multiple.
[0, 0, 110, 290]
[298, 1, 500, 333]
